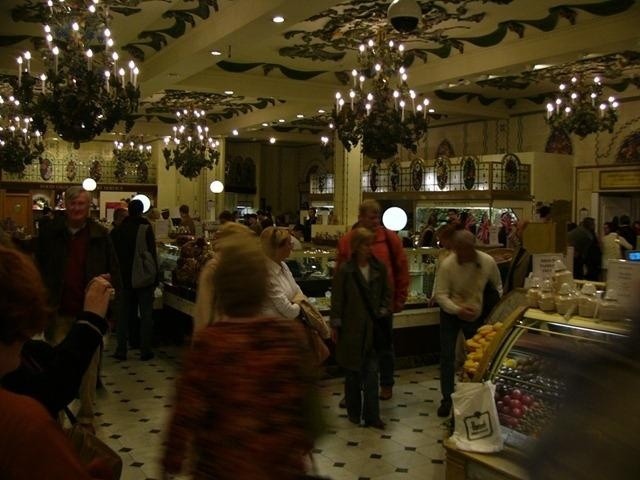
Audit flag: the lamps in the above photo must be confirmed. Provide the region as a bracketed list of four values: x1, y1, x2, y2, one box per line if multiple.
[0, 75, 45, 172]
[207, 182, 224, 210]
[545, 71, 619, 138]
[162, 104, 220, 180]
[111, 140, 153, 162]
[336, 32, 434, 161]
[15, 8, 141, 150]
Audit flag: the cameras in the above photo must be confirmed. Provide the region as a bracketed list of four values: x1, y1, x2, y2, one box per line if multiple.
[109, 288, 115, 301]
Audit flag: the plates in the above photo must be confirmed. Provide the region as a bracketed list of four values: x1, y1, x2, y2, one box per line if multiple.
[525, 258, 640, 324]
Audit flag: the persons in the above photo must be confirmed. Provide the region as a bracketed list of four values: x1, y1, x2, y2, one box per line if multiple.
[1, 248, 115, 480]
[36, 186, 117, 437]
[331, 228, 392, 429]
[159, 233, 319, 480]
[434, 231, 502, 417]
[0, 233, 114, 415]
[336, 198, 410, 408]
[1, 199, 640, 361]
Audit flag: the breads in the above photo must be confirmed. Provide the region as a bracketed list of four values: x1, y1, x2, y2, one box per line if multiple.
[525, 269, 623, 322]
[464, 323, 504, 368]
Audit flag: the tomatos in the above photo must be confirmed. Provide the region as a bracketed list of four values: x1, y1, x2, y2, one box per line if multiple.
[494, 382, 533, 430]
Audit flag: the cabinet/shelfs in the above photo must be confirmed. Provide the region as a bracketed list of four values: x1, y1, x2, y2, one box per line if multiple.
[155, 232, 448, 378]
[443, 307, 639, 480]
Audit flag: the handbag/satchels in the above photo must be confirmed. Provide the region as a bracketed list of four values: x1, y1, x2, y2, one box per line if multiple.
[61, 405, 122, 480]
[131, 224, 157, 289]
[372, 315, 393, 351]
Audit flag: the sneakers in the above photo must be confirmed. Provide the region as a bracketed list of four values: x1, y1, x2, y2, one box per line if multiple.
[113, 351, 127, 361]
[349, 414, 360, 424]
[365, 418, 387, 430]
[339, 398, 347, 408]
[379, 385, 392, 400]
[437, 397, 453, 417]
[140, 352, 155, 361]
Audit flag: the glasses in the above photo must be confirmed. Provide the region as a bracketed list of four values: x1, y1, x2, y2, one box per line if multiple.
[288, 241, 294, 250]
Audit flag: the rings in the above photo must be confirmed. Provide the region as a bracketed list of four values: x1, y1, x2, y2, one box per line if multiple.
[106, 290, 111, 293]
